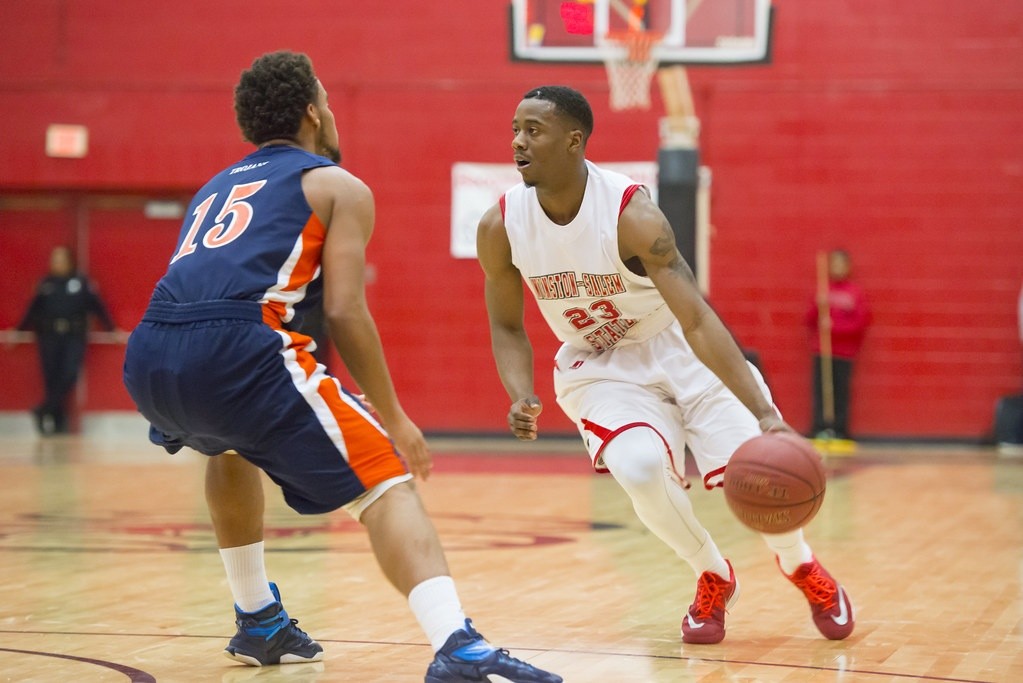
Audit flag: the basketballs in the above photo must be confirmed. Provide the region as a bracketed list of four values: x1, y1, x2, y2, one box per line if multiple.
[722, 431, 828, 535]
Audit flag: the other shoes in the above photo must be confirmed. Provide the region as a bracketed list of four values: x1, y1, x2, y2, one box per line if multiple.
[52, 420, 65, 434]
[32, 405, 44, 434]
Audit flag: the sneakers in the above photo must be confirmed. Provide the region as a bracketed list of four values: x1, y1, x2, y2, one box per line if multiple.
[777, 552, 854, 641]
[424, 618, 564, 683]
[223, 582, 333, 664]
[221, 662, 325, 683]
[681, 556, 742, 644]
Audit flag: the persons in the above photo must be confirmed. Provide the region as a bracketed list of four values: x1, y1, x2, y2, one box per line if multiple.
[12, 246, 115, 435]
[121, 51, 564, 683]
[811, 250, 862, 435]
[476, 85, 854, 642]
